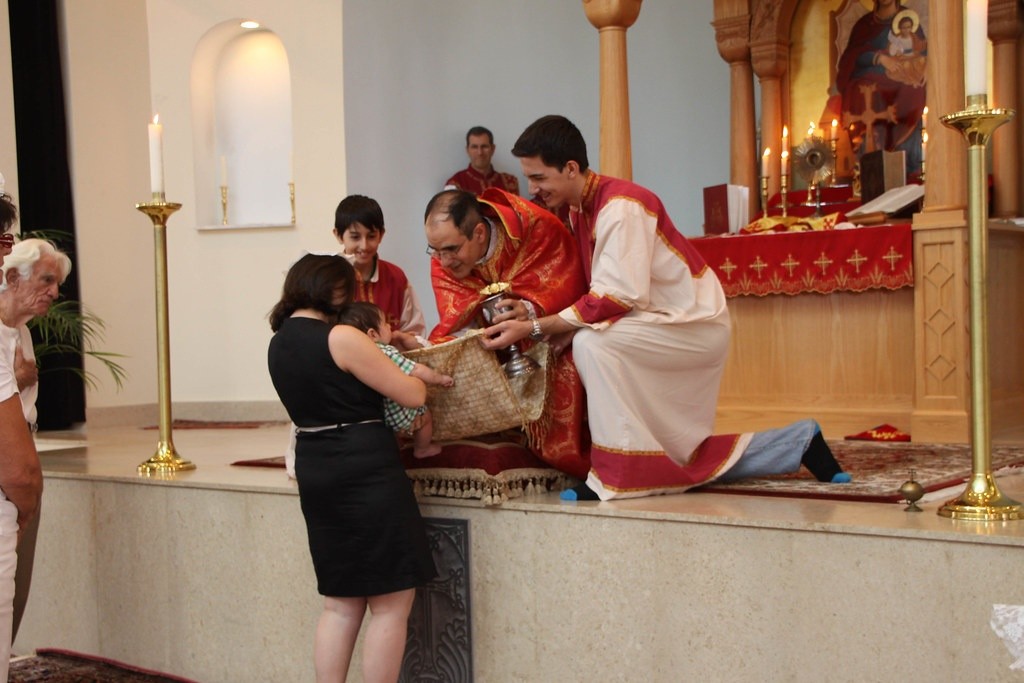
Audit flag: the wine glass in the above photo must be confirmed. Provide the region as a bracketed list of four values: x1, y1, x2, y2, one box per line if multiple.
[480, 292, 541, 379]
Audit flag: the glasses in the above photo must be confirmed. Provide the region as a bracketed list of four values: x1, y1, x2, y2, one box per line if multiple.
[426, 234, 468, 261]
[0, 234, 15, 248]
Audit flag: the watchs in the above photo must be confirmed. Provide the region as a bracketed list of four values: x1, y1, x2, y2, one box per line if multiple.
[529, 318, 544, 340]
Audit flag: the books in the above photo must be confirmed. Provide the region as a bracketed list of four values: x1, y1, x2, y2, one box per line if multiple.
[859, 150, 907, 206]
[845, 183, 925, 218]
[703, 183, 750, 235]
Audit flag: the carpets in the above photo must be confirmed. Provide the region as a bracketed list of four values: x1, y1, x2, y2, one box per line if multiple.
[231, 435, 1024, 503]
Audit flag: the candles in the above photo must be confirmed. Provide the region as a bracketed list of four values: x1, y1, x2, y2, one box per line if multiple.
[831, 118, 838, 152]
[780, 126, 789, 176]
[148, 114, 164, 192]
[963, 0, 988, 95]
[762, 147, 771, 177]
[219, 155, 227, 188]
[922, 107, 928, 162]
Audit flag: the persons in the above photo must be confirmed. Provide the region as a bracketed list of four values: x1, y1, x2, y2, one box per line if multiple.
[267, 254, 428, 683]
[446, 126, 520, 196]
[423, 187, 590, 478]
[331, 194, 428, 340]
[338, 302, 455, 458]
[478, 114, 853, 505]
[1, 194, 71, 683]
